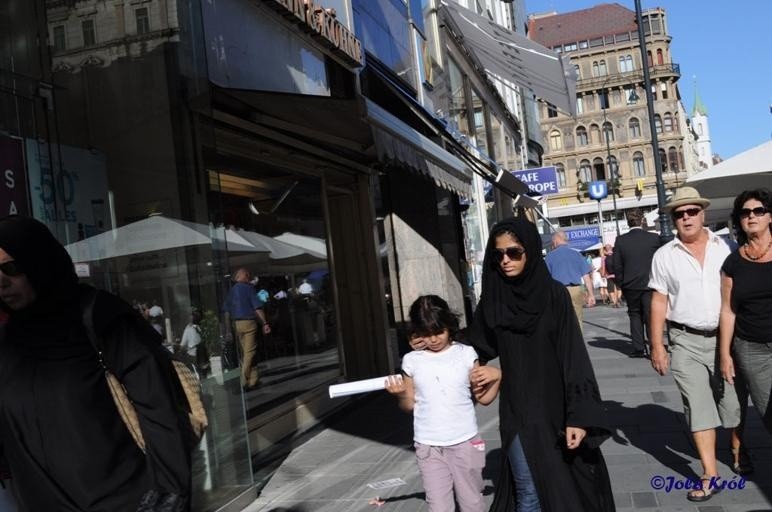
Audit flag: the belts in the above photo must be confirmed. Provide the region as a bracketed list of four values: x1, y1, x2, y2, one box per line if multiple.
[233, 319, 255, 321]
[564, 283, 580, 287]
[666, 320, 719, 338]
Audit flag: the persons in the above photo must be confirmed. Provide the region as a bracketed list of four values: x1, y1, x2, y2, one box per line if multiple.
[615, 208, 663, 358]
[718, 188, 772, 432]
[407, 217, 615, 512]
[1, 213, 194, 511]
[647, 185, 754, 501]
[391, 294, 502, 512]
[223, 267, 271, 394]
[544, 231, 596, 338]
[585, 244, 626, 308]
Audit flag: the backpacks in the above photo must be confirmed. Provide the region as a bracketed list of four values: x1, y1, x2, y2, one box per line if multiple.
[603, 254, 615, 274]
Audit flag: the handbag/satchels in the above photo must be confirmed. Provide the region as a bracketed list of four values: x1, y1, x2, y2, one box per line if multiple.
[81, 288, 209, 457]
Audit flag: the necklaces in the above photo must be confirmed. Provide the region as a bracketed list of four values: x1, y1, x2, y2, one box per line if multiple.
[743, 238, 772, 262]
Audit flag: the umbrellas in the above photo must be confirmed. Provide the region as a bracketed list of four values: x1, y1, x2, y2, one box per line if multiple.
[679, 141, 771, 226]
[63, 211, 330, 353]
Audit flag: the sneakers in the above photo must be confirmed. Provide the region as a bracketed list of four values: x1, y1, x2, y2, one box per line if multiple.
[602, 298, 625, 308]
[240, 381, 265, 391]
[628, 344, 668, 358]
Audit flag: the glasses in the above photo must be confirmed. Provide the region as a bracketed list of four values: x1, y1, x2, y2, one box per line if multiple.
[0, 260, 25, 277]
[490, 247, 526, 263]
[738, 207, 770, 218]
[673, 208, 702, 219]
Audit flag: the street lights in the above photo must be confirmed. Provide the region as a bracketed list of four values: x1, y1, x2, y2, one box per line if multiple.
[600, 74, 639, 237]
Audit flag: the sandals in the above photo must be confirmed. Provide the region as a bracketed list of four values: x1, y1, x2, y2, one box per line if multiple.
[686, 474, 724, 502]
[730, 445, 754, 475]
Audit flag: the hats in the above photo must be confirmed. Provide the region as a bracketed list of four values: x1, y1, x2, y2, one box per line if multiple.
[662, 186, 711, 213]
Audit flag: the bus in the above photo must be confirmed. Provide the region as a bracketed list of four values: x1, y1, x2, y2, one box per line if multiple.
[533, 195, 677, 288]
